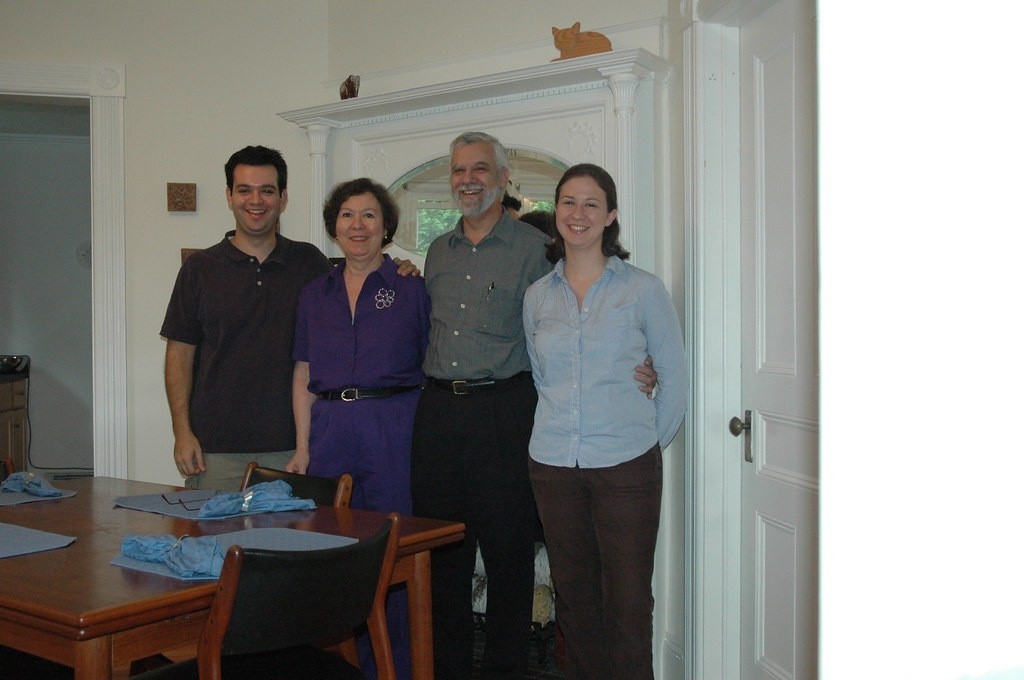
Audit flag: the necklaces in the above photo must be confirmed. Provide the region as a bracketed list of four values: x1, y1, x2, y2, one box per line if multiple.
[344, 268, 369, 310]
[577, 294, 583, 308]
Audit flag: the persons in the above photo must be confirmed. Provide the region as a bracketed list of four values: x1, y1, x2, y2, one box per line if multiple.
[418, 131, 690, 680]
[286, 177, 433, 680]
[160, 146, 422, 493]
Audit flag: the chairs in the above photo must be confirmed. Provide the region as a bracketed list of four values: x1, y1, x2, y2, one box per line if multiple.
[241, 462, 363, 674]
[131, 512, 403, 680]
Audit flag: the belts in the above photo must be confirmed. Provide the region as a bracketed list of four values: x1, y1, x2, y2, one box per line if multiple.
[320, 386, 416, 402]
[425, 378, 514, 395]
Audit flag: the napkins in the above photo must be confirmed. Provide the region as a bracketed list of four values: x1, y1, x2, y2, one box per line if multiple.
[1, 471, 62, 497]
[120, 530, 225, 578]
[199, 479, 321, 517]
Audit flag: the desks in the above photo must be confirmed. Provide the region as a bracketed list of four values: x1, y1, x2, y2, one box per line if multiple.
[0, 478, 467, 680]
